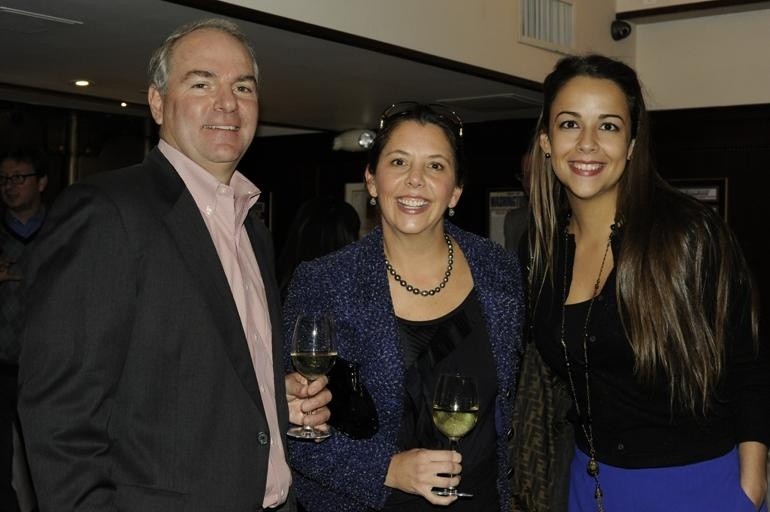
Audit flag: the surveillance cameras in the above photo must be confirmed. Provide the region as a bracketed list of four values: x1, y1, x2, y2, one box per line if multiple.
[612, 20, 631, 40]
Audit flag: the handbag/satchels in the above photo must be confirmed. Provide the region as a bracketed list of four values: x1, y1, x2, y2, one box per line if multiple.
[323, 356, 378, 440]
[513, 342, 575, 512]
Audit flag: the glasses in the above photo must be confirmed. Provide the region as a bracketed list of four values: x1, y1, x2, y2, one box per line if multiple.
[379, 102, 464, 141]
[0, 173, 39, 186]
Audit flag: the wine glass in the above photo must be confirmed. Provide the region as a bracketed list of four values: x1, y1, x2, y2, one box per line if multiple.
[430, 372, 481, 498]
[285, 310, 340, 440]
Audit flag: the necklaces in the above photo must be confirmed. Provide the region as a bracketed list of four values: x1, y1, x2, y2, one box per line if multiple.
[385, 234, 454, 296]
[561, 218, 615, 510]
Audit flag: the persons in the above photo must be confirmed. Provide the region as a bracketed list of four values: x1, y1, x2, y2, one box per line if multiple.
[516, 148, 534, 264]
[281, 102, 527, 512]
[17, 19, 333, 512]
[276, 197, 360, 303]
[517, 54, 770, 512]
[0, 146, 51, 512]
